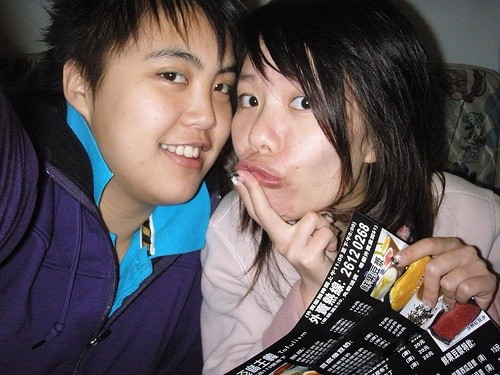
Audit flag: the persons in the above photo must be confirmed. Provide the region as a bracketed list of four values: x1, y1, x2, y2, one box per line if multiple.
[200, 0, 500, 375]
[0, 0, 248, 375]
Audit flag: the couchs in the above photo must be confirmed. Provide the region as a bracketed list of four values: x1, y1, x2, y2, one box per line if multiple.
[0, 47, 500, 196]
